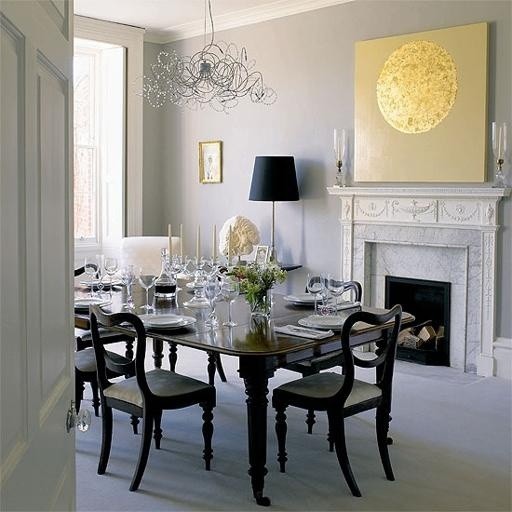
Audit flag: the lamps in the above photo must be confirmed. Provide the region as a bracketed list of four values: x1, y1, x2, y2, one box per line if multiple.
[132, 0, 278, 115]
[249, 156, 300, 265]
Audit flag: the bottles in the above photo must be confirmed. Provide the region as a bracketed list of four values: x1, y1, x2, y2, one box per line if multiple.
[154, 247, 179, 308]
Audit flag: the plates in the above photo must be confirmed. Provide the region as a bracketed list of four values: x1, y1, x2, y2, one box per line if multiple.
[283, 293, 328, 306]
[298, 315, 346, 330]
[80, 280, 123, 290]
[74, 298, 112, 311]
[136, 314, 197, 331]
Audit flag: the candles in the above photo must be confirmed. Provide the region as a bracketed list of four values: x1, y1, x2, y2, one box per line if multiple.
[168, 223, 172, 265]
[212, 224, 217, 266]
[180, 224, 185, 264]
[228, 224, 231, 266]
[196, 224, 200, 269]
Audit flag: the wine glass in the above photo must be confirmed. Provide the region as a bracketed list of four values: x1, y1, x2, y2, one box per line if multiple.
[121, 264, 136, 309]
[169, 257, 184, 293]
[203, 276, 219, 327]
[325, 273, 345, 318]
[85, 256, 102, 296]
[104, 256, 121, 294]
[307, 272, 326, 319]
[221, 277, 240, 327]
[138, 266, 156, 309]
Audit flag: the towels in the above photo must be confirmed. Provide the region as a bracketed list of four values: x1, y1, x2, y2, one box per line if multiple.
[275, 324, 334, 340]
[322, 300, 362, 311]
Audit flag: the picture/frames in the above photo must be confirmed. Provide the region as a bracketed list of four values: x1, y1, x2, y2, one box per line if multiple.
[198, 141, 222, 184]
[254, 246, 269, 265]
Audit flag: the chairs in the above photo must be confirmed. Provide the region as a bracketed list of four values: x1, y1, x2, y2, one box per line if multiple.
[304, 276, 362, 301]
[272, 304, 402, 498]
[75, 305, 140, 434]
[89, 304, 216, 492]
[74, 264, 135, 360]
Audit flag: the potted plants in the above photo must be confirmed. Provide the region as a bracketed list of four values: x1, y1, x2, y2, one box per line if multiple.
[225, 263, 288, 336]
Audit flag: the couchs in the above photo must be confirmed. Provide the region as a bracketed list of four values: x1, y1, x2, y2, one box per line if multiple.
[108, 237, 180, 275]
